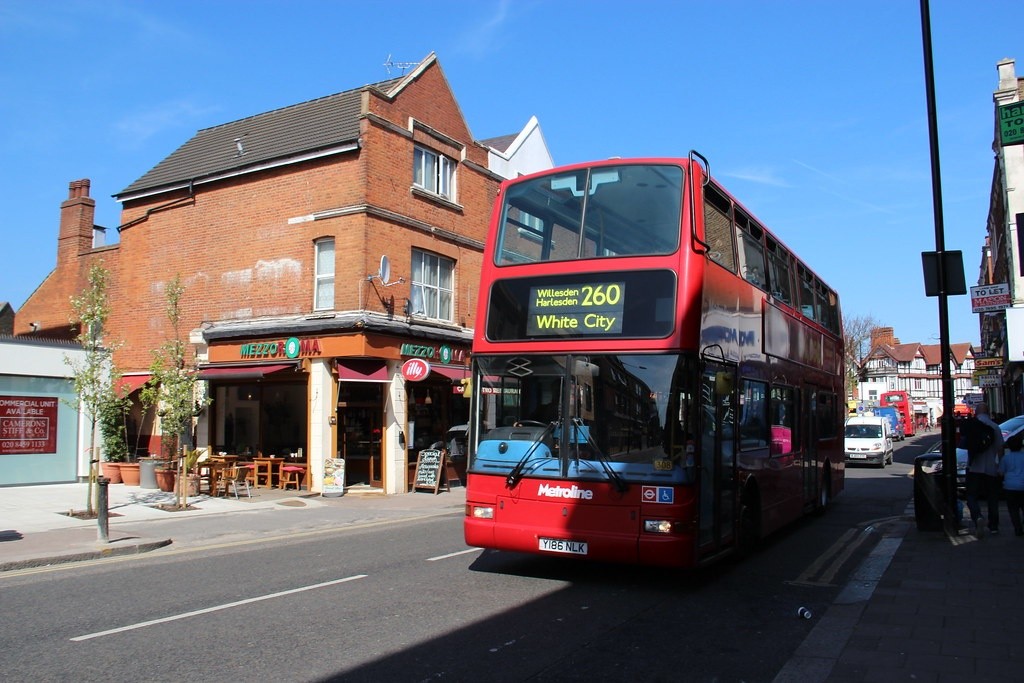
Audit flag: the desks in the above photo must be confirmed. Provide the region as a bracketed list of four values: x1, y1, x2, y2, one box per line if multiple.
[253, 458, 285, 490]
[197, 461, 230, 498]
[210, 455, 238, 485]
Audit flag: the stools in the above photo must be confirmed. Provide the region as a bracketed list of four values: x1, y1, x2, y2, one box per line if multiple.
[246, 465, 254, 486]
[281, 466, 305, 491]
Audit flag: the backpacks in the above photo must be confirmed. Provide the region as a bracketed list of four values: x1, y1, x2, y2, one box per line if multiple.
[960, 417, 995, 453]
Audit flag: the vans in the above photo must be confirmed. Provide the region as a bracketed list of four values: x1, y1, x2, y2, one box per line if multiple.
[871, 406, 904, 442]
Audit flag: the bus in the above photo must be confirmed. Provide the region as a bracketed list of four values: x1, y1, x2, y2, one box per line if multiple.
[880, 391, 917, 437]
[460, 150, 848, 571]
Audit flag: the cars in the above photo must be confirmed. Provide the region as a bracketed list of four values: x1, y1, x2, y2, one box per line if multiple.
[845, 415, 894, 468]
[930, 414, 1024, 501]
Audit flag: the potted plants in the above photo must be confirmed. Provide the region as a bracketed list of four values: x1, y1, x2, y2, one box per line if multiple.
[94, 394, 209, 497]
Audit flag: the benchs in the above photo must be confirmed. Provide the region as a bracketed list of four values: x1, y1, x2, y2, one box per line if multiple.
[767, 290, 814, 319]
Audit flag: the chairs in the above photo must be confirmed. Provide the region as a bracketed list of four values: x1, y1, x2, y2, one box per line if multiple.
[217, 466, 251, 500]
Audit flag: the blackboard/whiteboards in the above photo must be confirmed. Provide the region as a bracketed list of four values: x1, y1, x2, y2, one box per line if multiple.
[412, 448, 450, 495]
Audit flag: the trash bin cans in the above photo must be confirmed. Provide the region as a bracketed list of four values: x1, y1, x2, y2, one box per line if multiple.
[914, 453, 944, 532]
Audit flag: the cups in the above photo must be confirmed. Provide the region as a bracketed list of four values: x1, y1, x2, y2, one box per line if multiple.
[798, 607, 811, 618]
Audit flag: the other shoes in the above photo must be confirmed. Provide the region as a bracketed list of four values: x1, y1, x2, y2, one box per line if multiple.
[1015, 529, 1024, 536]
[975, 518, 985, 539]
[985, 526, 999, 534]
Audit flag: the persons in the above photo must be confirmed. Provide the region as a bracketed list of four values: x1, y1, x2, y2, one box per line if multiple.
[924, 418, 931, 432]
[996, 435, 1024, 539]
[513, 378, 563, 429]
[964, 403, 1004, 535]
